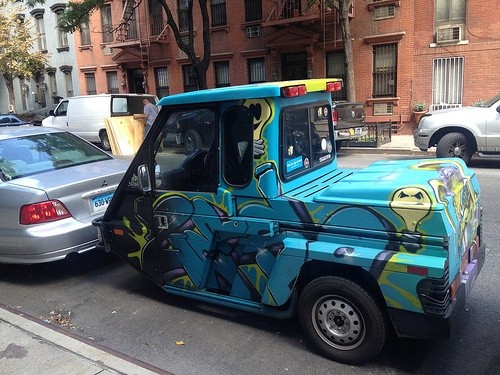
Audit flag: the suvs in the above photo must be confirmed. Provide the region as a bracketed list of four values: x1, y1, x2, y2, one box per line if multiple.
[412, 95, 500, 165]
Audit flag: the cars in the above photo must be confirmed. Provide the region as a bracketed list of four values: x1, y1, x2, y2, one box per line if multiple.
[0, 114, 35, 128]
[92, 79, 488, 365]
[0, 127, 132, 266]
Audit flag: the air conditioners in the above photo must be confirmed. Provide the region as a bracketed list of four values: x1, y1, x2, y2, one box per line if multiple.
[436, 25, 462, 43]
[246, 27, 262, 37]
[374, 103, 394, 115]
[373, 6, 395, 21]
[103, 47, 113, 55]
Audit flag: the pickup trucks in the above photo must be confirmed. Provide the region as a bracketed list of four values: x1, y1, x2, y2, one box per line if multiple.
[287, 99, 365, 155]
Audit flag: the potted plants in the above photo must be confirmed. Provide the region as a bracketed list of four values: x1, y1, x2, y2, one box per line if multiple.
[413, 100, 428, 124]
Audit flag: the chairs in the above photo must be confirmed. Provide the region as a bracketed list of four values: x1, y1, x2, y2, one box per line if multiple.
[202, 105, 254, 185]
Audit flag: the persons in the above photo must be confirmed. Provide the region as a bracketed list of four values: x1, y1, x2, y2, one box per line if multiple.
[133, 99, 163, 152]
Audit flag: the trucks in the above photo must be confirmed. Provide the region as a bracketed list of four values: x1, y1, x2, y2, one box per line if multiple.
[43, 93, 162, 151]
